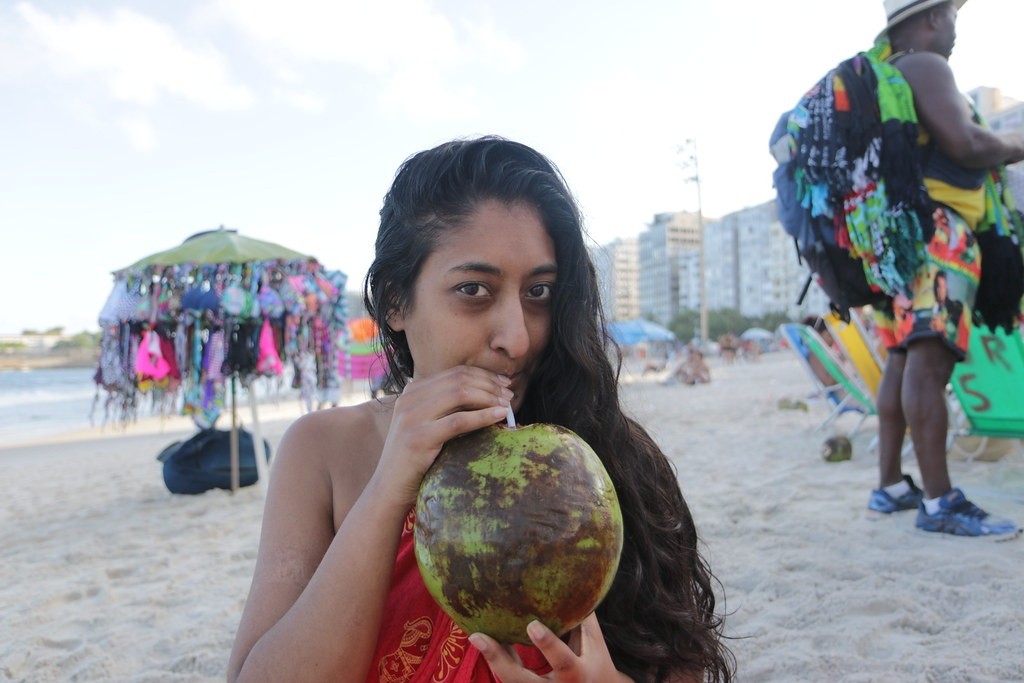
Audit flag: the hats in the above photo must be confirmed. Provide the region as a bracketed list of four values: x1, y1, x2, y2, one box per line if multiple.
[874, 0, 967, 44]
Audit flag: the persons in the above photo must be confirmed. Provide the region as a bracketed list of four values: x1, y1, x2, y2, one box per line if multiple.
[226, 135, 738, 683]
[866, 0, 1024, 538]
[800, 314, 846, 405]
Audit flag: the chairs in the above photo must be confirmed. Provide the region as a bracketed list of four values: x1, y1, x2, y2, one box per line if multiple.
[774, 304, 1024, 464]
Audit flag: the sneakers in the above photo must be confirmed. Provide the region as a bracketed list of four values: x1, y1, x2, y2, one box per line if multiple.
[868, 474, 922, 513]
[916, 487, 1017, 539]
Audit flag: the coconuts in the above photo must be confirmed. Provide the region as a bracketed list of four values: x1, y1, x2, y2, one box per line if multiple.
[822, 436, 852, 462]
[412, 422, 623, 645]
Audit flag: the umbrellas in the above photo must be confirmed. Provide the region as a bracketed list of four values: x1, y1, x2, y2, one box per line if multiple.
[739, 326, 774, 345]
[598, 316, 675, 377]
[110, 229, 317, 495]
